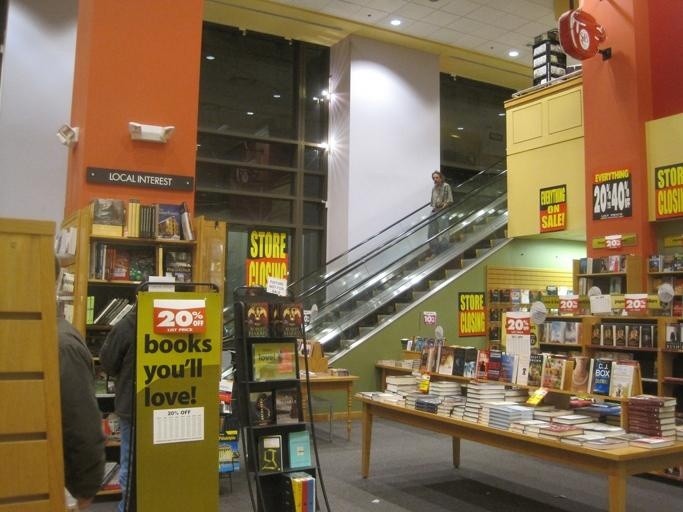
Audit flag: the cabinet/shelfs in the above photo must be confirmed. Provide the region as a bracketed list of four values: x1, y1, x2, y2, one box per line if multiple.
[217, 398, 241, 497]
[58, 201, 226, 499]
[530, 254, 683, 486]
[232, 285, 332, 512]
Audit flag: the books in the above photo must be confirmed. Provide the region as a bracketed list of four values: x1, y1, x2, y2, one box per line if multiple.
[276, 389, 298, 425]
[246, 303, 269, 338]
[251, 342, 296, 382]
[86, 200, 191, 490]
[579, 256, 683, 291]
[358, 335, 683, 451]
[280, 472, 316, 512]
[259, 435, 283, 473]
[288, 431, 311, 469]
[489, 285, 683, 351]
[249, 391, 273, 427]
[281, 304, 303, 337]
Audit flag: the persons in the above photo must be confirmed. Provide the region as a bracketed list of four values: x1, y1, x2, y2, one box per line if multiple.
[55, 255, 104, 508]
[430, 171, 454, 255]
[99, 282, 148, 512]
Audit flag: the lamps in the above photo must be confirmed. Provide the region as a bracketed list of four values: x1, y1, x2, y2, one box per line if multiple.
[56, 125, 80, 145]
[128, 121, 175, 144]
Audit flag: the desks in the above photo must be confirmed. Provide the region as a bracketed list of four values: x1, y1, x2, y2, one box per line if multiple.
[354, 391, 682, 512]
[301, 367, 360, 442]
[375, 360, 474, 392]
